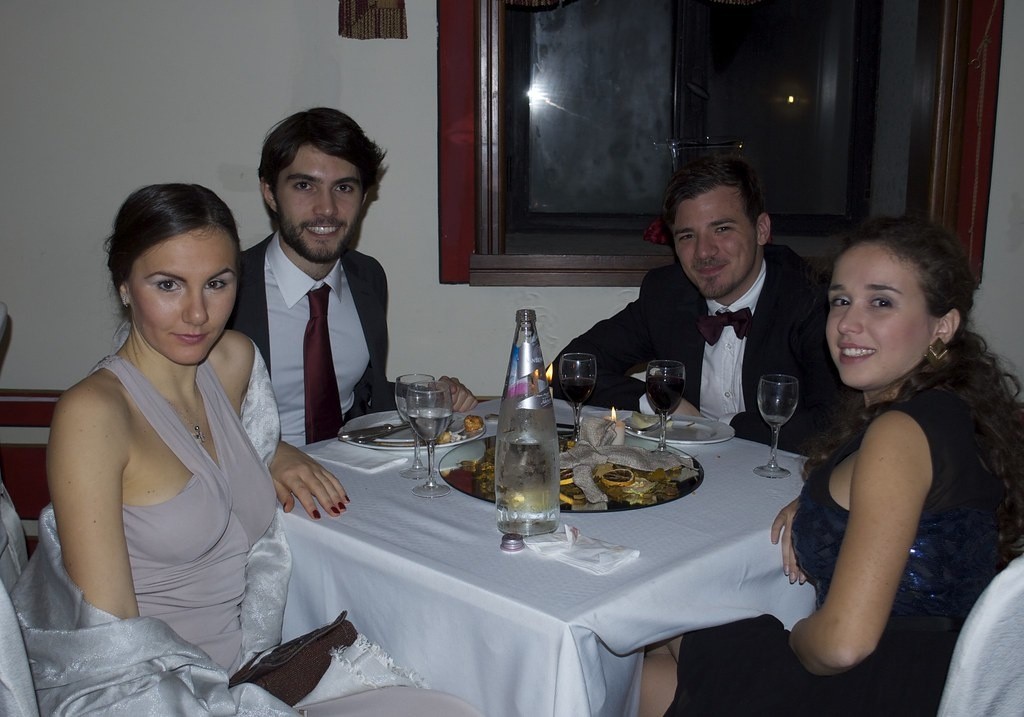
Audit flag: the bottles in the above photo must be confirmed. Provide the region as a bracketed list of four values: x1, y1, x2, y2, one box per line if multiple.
[494, 310, 560, 534]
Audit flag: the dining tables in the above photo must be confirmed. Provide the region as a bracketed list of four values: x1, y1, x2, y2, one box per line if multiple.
[276, 397, 814, 717]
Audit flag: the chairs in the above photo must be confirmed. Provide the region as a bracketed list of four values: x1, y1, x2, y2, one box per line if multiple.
[936, 558, 1023, 717]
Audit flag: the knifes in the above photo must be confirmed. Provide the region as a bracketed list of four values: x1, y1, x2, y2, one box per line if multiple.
[355, 425, 408, 444]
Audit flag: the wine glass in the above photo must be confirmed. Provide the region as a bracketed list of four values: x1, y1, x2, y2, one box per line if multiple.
[408, 382, 452, 497]
[560, 353, 596, 442]
[753, 373, 799, 478]
[646, 360, 686, 455]
[394, 373, 436, 480]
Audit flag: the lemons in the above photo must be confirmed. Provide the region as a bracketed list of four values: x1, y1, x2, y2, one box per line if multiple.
[558, 468, 633, 505]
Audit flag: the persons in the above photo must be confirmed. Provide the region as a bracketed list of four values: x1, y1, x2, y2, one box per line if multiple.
[636, 211, 1024, 717]
[223, 107, 475, 448]
[546, 146, 867, 471]
[46, 182, 487, 717]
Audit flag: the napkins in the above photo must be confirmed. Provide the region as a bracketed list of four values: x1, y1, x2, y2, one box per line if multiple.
[306, 440, 409, 475]
[523, 524, 640, 576]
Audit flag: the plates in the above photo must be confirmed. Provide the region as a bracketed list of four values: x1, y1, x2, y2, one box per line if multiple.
[337, 408, 487, 449]
[620, 415, 735, 443]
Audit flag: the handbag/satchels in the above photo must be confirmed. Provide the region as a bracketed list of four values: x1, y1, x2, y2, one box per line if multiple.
[227, 610, 358, 705]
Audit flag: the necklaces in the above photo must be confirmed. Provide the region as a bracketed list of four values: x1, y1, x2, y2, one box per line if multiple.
[162, 384, 206, 444]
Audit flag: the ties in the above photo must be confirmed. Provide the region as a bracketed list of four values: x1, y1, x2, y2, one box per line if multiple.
[303, 285, 344, 444]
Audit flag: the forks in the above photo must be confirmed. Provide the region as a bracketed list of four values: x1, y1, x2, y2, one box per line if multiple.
[337, 418, 455, 441]
[623, 415, 673, 435]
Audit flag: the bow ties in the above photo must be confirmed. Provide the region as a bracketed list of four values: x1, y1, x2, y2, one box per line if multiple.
[695, 307, 753, 346]
